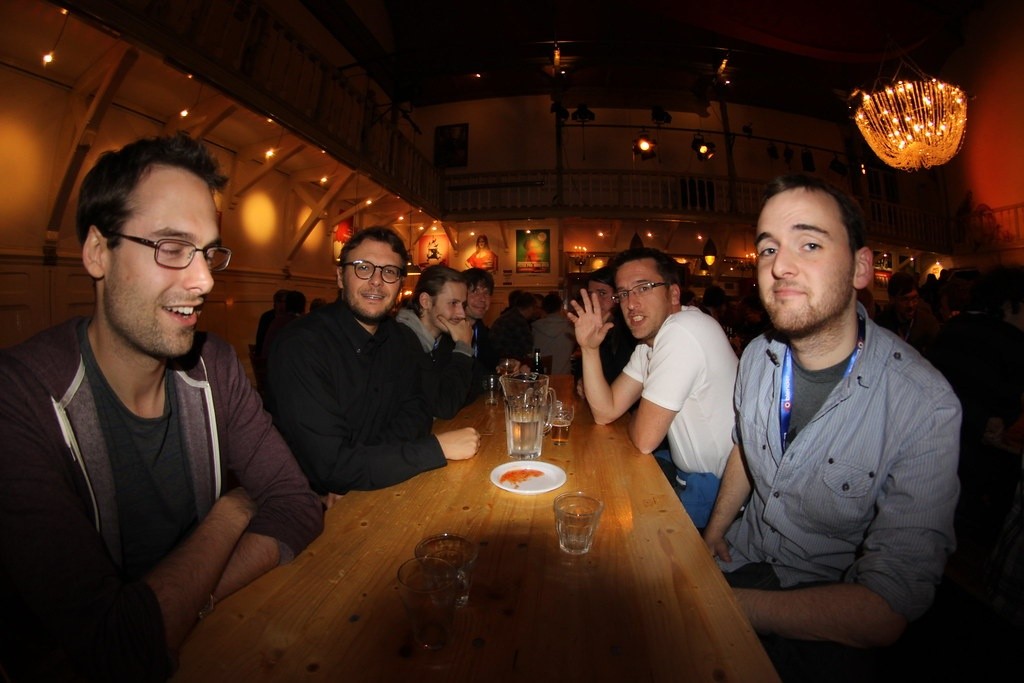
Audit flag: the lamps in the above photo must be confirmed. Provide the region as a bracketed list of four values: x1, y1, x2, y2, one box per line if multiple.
[846, 34, 967, 174]
[766, 141, 850, 176]
[628, 227, 643, 250]
[632, 104, 718, 162]
[555, 101, 594, 122]
[702, 236, 717, 266]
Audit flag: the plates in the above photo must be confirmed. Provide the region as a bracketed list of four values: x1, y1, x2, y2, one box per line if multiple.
[490, 460, 566, 495]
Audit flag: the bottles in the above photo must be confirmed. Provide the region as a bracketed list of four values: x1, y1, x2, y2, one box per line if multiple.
[532, 348, 546, 375]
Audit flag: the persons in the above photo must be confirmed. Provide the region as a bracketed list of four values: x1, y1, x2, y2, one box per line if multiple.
[0, 131, 325, 683]
[461, 268, 521, 392]
[394, 265, 481, 420]
[266, 225, 482, 508]
[875, 270, 1024, 558]
[465, 234, 498, 273]
[489, 290, 576, 374]
[254, 289, 306, 364]
[567, 246, 739, 528]
[701, 174, 962, 649]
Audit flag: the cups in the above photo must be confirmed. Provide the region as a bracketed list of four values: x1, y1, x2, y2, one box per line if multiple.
[554, 491, 604, 555]
[481, 374, 502, 408]
[396, 556, 461, 654]
[548, 404, 575, 446]
[414, 533, 480, 609]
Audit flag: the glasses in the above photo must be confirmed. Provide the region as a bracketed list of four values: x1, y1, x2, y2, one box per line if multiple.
[610, 281, 672, 304]
[100, 228, 231, 272]
[343, 260, 407, 284]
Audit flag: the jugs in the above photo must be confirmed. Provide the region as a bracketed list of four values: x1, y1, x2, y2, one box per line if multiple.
[498, 373, 557, 460]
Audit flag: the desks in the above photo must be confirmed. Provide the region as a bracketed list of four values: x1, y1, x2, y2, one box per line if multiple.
[172, 375, 783, 683]
